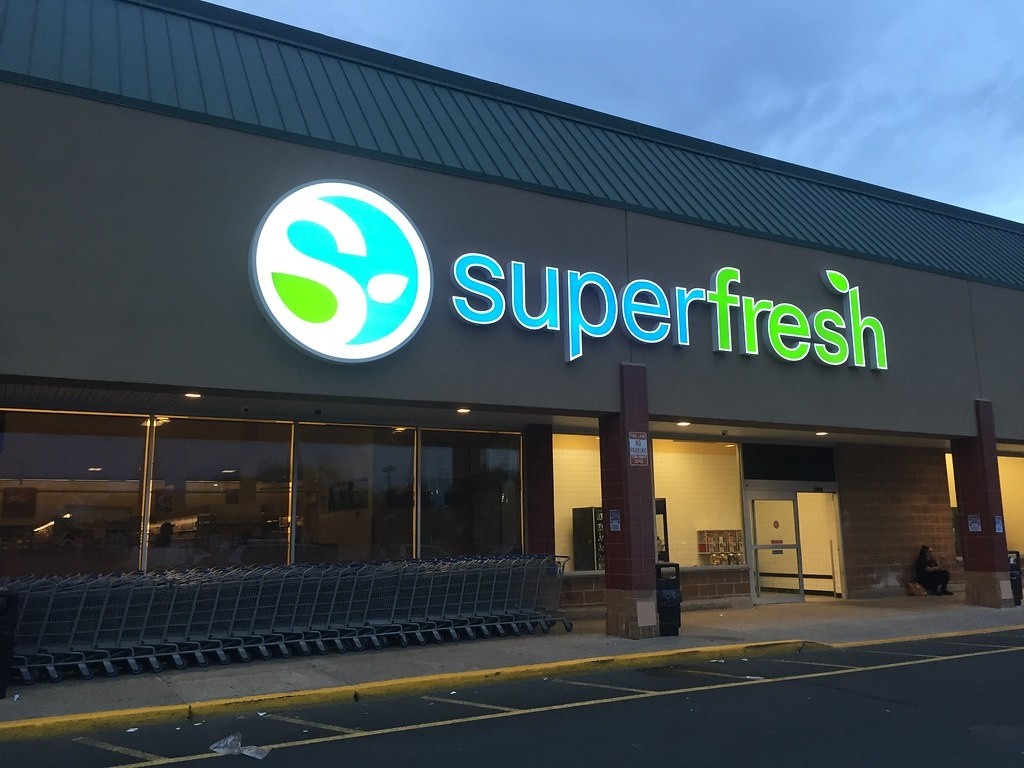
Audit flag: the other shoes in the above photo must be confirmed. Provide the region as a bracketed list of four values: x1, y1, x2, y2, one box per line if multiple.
[941, 589, 953, 595]
[936, 590, 941, 597]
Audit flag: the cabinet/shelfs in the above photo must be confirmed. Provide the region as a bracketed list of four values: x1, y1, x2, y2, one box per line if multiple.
[697, 530, 744, 566]
[0, 503, 301, 555]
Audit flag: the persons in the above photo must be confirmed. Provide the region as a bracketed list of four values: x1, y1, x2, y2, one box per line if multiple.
[155, 522, 176, 547]
[916, 545, 954, 596]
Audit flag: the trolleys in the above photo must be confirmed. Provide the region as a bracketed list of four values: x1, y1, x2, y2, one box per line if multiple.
[0, 554, 575, 687]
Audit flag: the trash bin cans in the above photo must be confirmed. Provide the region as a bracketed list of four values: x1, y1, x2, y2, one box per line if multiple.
[1007, 551, 1023, 606]
[655, 562, 681, 637]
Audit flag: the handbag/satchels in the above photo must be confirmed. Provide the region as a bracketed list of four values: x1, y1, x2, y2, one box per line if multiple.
[908, 580, 928, 595]
